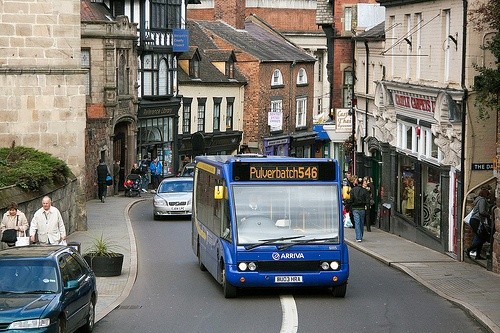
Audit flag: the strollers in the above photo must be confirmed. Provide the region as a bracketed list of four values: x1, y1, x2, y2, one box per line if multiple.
[125, 174, 143, 196]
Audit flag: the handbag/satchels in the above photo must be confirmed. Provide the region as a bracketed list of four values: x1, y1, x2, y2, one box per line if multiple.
[370, 194, 374, 205]
[59, 239, 68, 246]
[482, 217, 492, 242]
[343, 212, 353, 227]
[105, 173, 113, 185]
[463, 210, 473, 224]
[1, 229, 17, 247]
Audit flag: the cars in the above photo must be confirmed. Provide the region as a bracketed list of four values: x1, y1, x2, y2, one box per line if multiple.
[153, 177, 194, 220]
[0, 245, 97, 333]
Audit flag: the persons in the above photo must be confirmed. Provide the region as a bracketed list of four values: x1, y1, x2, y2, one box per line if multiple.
[0, 201, 29, 250]
[29, 195, 66, 245]
[167, 184, 174, 191]
[183, 157, 189, 167]
[139, 155, 149, 193]
[221, 194, 268, 239]
[341, 170, 376, 232]
[39, 262, 63, 292]
[464, 185, 496, 259]
[125, 180, 138, 192]
[183, 183, 193, 190]
[150, 155, 162, 191]
[402, 175, 414, 217]
[131, 163, 142, 196]
[113, 160, 120, 195]
[95, 159, 111, 203]
[346, 177, 371, 242]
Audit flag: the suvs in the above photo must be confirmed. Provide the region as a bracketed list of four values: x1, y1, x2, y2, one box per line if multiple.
[180, 164, 194, 177]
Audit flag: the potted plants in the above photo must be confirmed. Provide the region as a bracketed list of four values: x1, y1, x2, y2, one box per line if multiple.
[81, 233, 125, 277]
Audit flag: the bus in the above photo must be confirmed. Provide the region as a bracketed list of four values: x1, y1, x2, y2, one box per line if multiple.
[191, 153, 350, 298]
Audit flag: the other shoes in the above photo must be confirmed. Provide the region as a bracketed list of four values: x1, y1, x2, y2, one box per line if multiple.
[464, 249, 471, 258]
[356, 239, 362, 241]
[475, 255, 486, 260]
[367, 227, 371, 232]
[101, 196, 105, 202]
